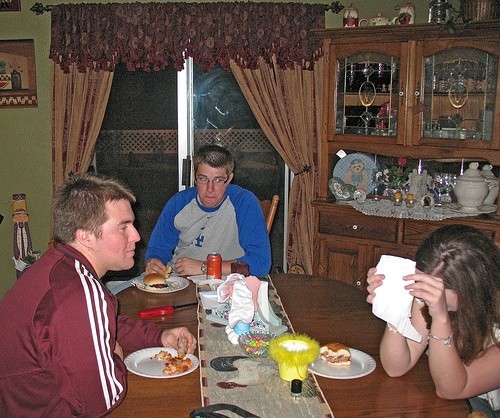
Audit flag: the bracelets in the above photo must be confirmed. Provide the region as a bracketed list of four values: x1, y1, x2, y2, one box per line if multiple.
[428, 331, 454, 348]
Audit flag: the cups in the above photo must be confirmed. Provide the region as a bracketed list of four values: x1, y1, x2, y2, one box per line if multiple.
[343, 7, 359, 28]
[398, 3, 415, 24]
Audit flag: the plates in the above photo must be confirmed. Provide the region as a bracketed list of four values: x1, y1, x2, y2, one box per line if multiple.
[308, 345, 376, 379]
[123, 346, 200, 378]
[136, 277, 189, 294]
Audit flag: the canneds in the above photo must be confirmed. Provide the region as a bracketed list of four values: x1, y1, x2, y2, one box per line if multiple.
[206, 253, 223, 279]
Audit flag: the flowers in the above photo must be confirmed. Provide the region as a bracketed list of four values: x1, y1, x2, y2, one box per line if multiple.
[378, 157, 411, 190]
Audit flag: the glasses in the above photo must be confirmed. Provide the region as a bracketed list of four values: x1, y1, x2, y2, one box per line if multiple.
[194, 171, 232, 186]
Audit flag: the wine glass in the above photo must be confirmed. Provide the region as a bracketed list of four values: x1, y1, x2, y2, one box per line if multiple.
[433, 172, 456, 203]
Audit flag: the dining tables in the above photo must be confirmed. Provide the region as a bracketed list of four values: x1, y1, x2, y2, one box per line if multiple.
[107, 273, 469, 418]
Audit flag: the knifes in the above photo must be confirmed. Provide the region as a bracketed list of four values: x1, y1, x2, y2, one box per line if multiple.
[139, 303, 196, 318]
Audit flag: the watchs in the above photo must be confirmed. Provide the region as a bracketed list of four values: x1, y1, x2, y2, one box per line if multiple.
[200, 261, 207, 274]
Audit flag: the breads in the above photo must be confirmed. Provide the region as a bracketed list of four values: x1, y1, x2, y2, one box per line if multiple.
[154, 338, 191, 374]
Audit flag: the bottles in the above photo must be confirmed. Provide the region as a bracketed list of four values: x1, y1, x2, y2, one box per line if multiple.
[392, 192, 415, 208]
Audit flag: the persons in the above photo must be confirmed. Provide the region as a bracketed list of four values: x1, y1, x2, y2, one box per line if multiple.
[144, 145, 272, 277]
[1, 173, 197, 418]
[366, 224, 500, 418]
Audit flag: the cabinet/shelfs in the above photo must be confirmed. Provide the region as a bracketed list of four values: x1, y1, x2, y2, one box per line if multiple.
[311, 20, 500, 295]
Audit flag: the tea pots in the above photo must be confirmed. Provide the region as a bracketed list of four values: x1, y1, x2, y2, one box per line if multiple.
[359, 13, 398, 26]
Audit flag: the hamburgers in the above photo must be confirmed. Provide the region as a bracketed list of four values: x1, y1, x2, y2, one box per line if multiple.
[144, 266, 173, 290]
[318, 343, 351, 366]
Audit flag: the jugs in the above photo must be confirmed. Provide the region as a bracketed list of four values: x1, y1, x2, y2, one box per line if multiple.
[451, 161, 499, 211]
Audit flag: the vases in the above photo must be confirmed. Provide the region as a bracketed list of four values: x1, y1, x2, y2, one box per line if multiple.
[387, 187, 408, 202]
